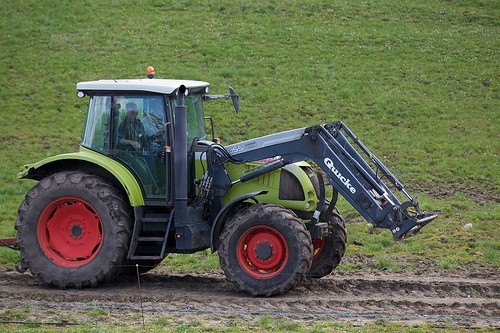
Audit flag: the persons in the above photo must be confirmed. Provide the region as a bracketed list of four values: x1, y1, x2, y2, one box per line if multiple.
[140, 100, 168, 157]
[115, 100, 162, 193]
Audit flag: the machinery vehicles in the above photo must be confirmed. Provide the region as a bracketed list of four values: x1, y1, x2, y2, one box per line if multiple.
[13, 66, 437, 297]
